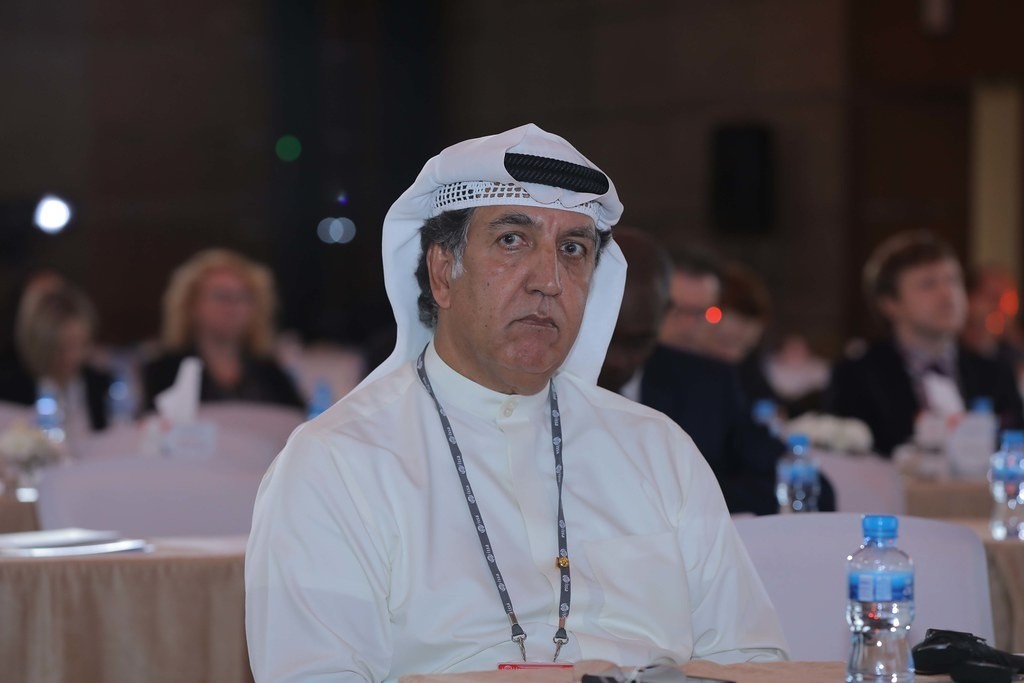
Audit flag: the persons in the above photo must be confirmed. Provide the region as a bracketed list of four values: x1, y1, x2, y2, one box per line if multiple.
[0, 273, 116, 433]
[598, 224, 1024, 516]
[244, 122, 790, 683]
[125, 247, 309, 419]
[828, 230, 1024, 461]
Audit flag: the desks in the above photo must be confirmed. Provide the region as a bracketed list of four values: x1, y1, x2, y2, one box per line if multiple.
[0, 533, 257, 683]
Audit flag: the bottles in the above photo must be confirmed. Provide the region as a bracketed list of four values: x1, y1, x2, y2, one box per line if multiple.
[986, 429, 1024, 542]
[306, 377, 332, 419]
[843, 509, 915, 683]
[776, 436, 835, 514]
[754, 400, 779, 439]
[947, 398, 998, 477]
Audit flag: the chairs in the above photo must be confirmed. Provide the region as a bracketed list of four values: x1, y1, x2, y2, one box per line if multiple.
[39, 344, 994, 660]
